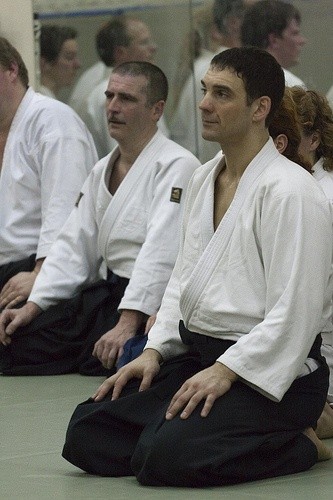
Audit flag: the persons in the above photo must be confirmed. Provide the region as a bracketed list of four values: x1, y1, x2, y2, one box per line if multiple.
[60, 47, 332, 487]
[84, 16, 170, 158]
[0, 36, 99, 313]
[70, 62, 114, 132]
[195, 0, 258, 165]
[169, 4, 227, 148]
[39, 26, 81, 100]
[291, 86, 333, 439]
[268, 86, 315, 176]
[0, 62, 201, 378]
[241, 1, 308, 93]
